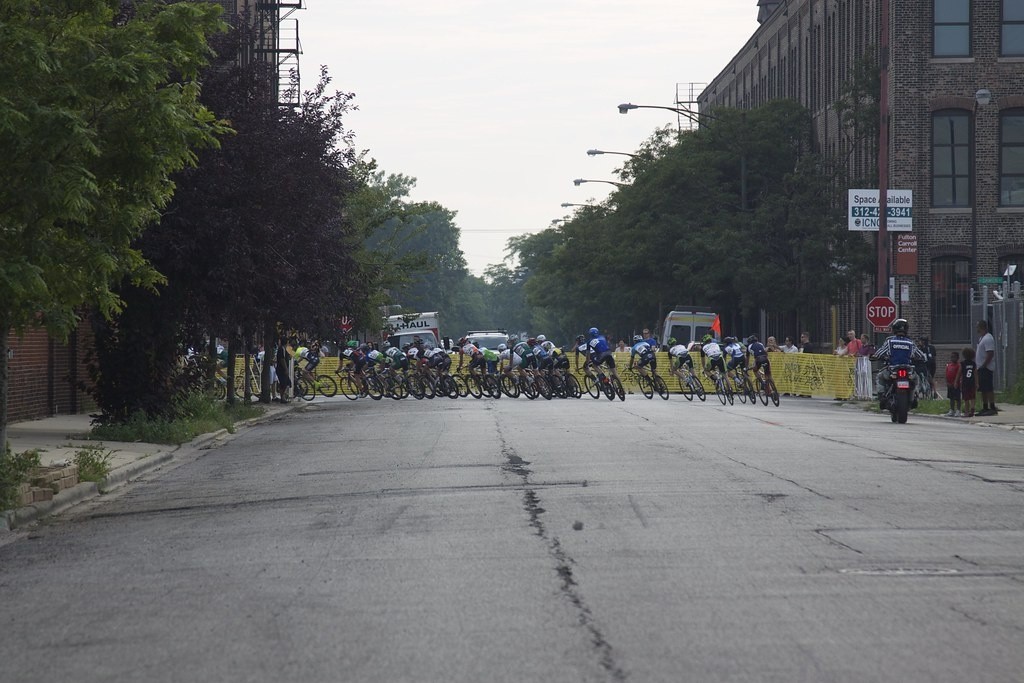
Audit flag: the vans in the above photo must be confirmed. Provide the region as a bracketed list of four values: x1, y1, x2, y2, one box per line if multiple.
[654, 305, 722, 352]
[464, 328, 509, 355]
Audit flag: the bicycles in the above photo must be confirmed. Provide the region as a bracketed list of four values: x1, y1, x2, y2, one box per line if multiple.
[729, 366, 757, 405]
[745, 363, 780, 407]
[666, 363, 707, 401]
[201, 360, 628, 401]
[702, 367, 735, 406]
[622, 362, 670, 401]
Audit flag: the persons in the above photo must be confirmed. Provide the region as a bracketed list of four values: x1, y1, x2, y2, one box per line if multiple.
[194, 335, 570, 403]
[615, 339, 629, 352]
[778, 336, 798, 396]
[574, 327, 626, 394]
[953, 347, 979, 416]
[668, 337, 700, 386]
[642, 328, 657, 351]
[833, 329, 877, 401]
[765, 336, 782, 352]
[974, 320, 997, 416]
[912, 335, 939, 400]
[798, 332, 815, 398]
[629, 335, 665, 393]
[700, 331, 779, 399]
[874, 318, 927, 407]
[946, 352, 963, 417]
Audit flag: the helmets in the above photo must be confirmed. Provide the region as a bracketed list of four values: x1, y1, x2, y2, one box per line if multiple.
[347, 340, 357, 347]
[892, 318, 909, 334]
[667, 337, 676, 344]
[747, 335, 758, 342]
[402, 342, 411, 349]
[415, 338, 424, 345]
[360, 343, 368, 350]
[588, 327, 599, 335]
[526, 338, 536, 345]
[472, 342, 479, 348]
[457, 337, 468, 346]
[498, 343, 507, 351]
[702, 334, 712, 342]
[507, 334, 519, 343]
[537, 335, 546, 341]
[576, 335, 585, 342]
[723, 337, 732, 343]
[633, 335, 643, 340]
[382, 341, 391, 348]
[424, 341, 434, 349]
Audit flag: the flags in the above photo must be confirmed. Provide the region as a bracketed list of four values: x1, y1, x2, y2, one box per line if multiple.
[712, 315, 721, 334]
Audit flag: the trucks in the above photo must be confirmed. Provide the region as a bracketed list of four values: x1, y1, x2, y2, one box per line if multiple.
[380, 309, 442, 350]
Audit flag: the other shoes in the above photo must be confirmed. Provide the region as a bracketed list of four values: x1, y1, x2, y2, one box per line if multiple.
[945, 407, 997, 417]
[281, 398, 289, 404]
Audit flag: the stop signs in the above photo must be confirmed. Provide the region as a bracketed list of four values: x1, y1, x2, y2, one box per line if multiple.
[865, 296, 898, 328]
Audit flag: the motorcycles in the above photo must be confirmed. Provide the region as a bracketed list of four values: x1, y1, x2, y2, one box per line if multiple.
[868, 352, 932, 425]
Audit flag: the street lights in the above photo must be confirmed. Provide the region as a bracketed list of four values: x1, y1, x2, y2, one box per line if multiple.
[615, 102, 768, 346]
[584, 148, 699, 305]
[967, 88, 996, 300]
